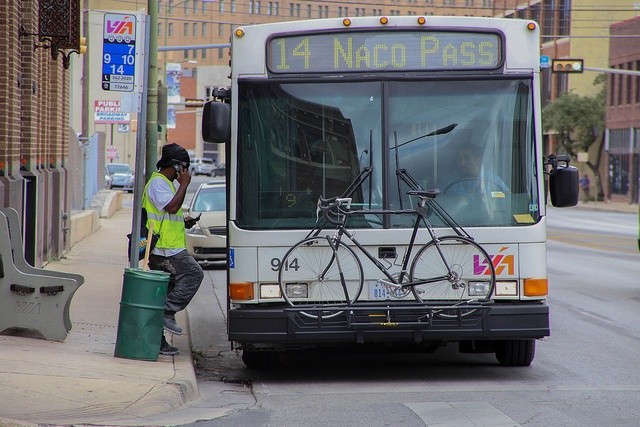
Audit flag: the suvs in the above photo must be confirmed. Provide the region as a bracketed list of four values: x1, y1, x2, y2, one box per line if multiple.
[189, 158, 215, 176]
[105, 163, 135, 193]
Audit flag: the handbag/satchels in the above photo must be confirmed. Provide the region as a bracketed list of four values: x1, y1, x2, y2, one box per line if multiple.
[126, 178, 171, 263]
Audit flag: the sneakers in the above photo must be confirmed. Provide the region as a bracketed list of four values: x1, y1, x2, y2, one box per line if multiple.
[163, 314, 183, 336]
[159, 338, 179, 356]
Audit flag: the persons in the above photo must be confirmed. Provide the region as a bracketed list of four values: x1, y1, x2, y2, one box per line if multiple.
[437, 143, 512, 195]
[139, 143, 204, 355]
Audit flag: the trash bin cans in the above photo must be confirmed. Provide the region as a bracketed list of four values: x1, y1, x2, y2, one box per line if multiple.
[113, 268, 171, 361]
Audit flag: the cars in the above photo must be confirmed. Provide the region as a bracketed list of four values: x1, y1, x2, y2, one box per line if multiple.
[211, 163, 226, 178]
[185, 180, 227, 269]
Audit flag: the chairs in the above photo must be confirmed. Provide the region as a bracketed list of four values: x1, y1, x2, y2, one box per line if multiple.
[0, 206, 86, 343]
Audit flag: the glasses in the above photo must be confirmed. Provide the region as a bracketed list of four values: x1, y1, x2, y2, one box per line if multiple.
[170, 158, 191, 170]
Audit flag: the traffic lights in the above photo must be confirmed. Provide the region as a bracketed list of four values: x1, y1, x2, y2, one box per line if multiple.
[552, 59, 583, 73]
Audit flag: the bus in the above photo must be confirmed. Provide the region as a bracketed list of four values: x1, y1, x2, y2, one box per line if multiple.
[202, 14, 579, 371]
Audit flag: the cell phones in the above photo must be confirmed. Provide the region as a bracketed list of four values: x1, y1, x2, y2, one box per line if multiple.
[173, 163, 183, 174]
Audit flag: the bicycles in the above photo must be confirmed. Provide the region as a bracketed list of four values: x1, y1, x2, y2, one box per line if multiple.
[279, 187, 495, 322]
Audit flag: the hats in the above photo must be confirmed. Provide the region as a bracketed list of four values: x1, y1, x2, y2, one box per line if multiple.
[161, 143, 190, 163]
